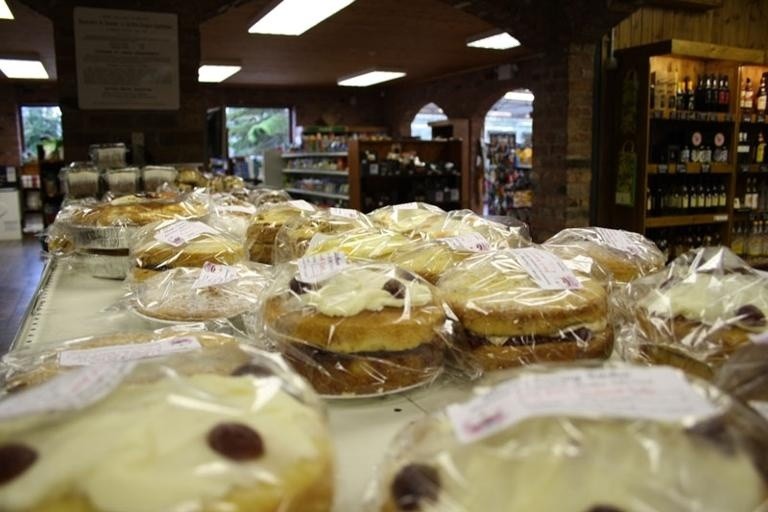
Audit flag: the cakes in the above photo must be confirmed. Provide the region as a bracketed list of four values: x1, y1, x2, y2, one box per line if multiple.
[375, 359, 768, 512]
[1, 170, 768, 400]
[0, 326, 334, 512]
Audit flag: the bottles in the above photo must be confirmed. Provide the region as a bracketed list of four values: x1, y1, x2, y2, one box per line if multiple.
[293, 126, 385, 149]
[644, 63, 767, 266]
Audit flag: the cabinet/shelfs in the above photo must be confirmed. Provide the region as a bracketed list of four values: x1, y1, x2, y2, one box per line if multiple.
[261, 120, 532, 241]
[20, 142, 63, 253]
[610, 39, 767, 272]
